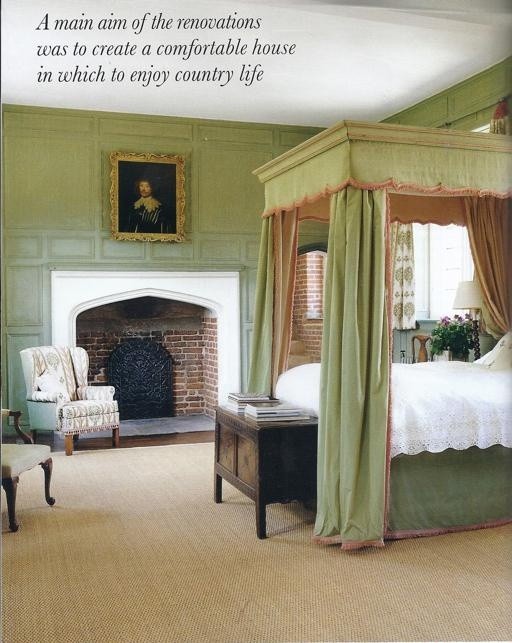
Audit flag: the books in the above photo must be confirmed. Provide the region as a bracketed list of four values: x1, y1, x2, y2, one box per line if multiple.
[227, 392, 312, 423]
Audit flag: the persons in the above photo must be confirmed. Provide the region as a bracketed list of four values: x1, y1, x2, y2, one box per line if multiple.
[127, 178, 172, 233]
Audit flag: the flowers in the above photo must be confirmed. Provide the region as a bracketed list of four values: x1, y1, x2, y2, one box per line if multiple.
[429, 311, 473, 361]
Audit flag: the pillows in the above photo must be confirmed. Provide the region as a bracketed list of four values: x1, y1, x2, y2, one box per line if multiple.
[481, 330, 512, 369]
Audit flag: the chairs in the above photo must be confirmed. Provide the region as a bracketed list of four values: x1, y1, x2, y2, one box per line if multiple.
[410, 334, 432, 364]
[18, 343, 122, 457]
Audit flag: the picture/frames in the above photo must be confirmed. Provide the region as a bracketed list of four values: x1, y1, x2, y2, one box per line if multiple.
[108, 148, 188, 246]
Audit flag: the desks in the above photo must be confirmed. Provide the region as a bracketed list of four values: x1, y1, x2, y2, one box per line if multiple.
[213, 407, 320, 539]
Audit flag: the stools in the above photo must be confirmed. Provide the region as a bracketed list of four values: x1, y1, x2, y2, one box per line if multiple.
[0, 406, 55, 528]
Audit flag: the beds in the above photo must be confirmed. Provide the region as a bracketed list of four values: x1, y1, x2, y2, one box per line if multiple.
[275, 354, 512, 546]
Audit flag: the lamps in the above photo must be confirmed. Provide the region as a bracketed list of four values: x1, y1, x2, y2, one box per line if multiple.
[451, 280, 483, 360]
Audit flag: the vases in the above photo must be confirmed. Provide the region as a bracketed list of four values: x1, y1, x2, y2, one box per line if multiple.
[435, 350, 464, 362]
